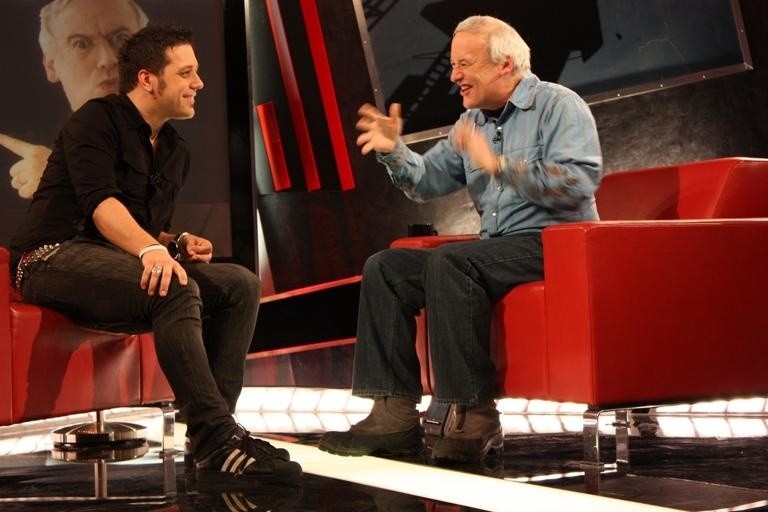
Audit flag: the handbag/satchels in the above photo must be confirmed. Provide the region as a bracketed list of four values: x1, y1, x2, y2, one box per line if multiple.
[138, 244, 169, 260]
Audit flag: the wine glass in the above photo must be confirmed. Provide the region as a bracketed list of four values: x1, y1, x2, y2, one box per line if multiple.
[152, 266, 162, 272]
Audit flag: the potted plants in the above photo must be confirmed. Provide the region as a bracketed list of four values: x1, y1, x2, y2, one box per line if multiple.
[0, 0, 258, 277]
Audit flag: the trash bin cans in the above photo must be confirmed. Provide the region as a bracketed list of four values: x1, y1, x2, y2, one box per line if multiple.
[167, 232, 188, 263]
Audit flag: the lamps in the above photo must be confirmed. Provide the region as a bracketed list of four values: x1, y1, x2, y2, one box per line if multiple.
[387, 152, 768, 477]
[3, 246, 182, 457]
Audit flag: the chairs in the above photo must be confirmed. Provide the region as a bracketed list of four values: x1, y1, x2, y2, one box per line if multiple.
[185, 427, 303, 489]
[318, 396, 422, 458]
[430, 398, 504, 465]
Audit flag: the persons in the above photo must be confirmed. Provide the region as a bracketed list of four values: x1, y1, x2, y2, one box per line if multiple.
[8, 24, 306, 482]
[0, 2, 151, 204]
[318, 14, 607, 467]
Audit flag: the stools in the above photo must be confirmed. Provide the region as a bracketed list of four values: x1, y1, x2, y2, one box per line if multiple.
[14, 244, 59, 292]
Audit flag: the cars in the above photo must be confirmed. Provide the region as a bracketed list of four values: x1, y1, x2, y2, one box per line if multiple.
[408, 222, 437, 236]
